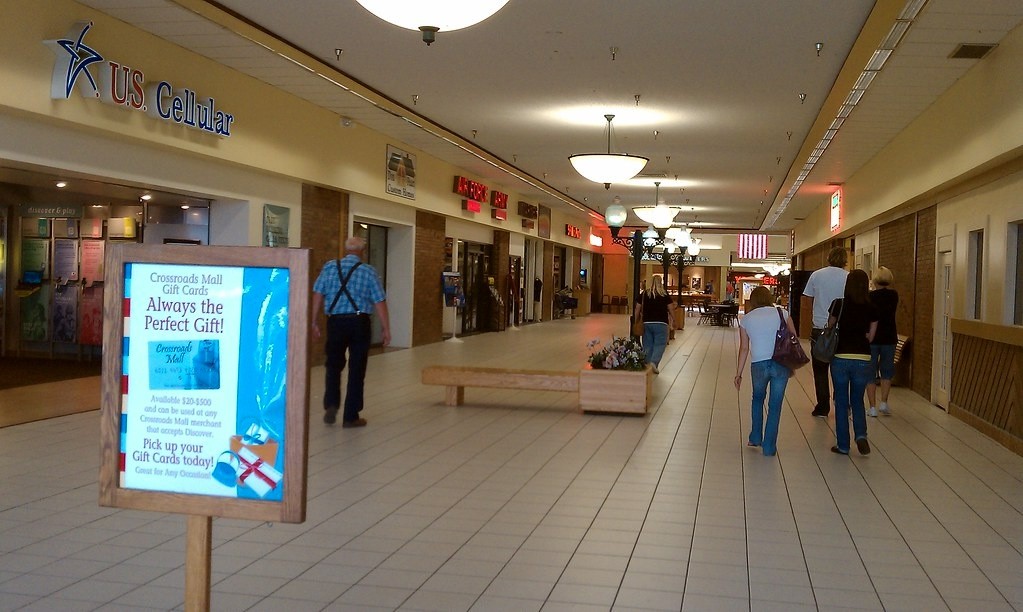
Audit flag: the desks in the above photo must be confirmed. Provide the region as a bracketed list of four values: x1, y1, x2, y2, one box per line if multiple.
[708, 305, 739, 326]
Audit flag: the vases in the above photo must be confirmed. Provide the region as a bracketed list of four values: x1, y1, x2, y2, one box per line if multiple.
[580, 363, 652, 418]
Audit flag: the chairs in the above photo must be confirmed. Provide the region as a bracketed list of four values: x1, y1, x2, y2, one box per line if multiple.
[698, 298, 740, 328]
[597, 295, 629, 315]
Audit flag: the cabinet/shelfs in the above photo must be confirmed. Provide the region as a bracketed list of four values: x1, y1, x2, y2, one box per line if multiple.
[573, 289, 592, 317]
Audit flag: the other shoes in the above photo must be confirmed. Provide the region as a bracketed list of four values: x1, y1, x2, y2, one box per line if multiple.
[343, 418, 368, 427]
[831, 446, 848, 454]
[879, 402, 892, 415]
[324, 407, 338, 424]
[650, 362, 660, 374]
[812, 409, 829, 417]
[857, 438, 870, 455]
[747, 441, 756, 446]
[867, 407, 877, 416]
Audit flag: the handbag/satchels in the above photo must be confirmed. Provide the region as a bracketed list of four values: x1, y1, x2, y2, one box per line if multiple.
[633, 313, 645, 336]
[814, 297, 844, 362]
[772, 306, 810, 369]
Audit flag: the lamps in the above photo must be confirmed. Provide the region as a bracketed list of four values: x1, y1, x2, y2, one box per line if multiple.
[632, 181, 681, 223]
[568, 115, 649, 191]
[354, 0, 511, 46]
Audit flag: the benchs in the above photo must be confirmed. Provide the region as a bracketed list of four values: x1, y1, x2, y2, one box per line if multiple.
[875, 334, 910, 384]
[421, 366, 581, 407]
[688, 294, 715, 311]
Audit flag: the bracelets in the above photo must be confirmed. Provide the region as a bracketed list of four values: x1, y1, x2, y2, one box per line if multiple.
[735, 376, 742, 378]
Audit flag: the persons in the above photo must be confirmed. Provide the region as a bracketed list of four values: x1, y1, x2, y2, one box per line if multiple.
[802, 247, 851, 418]
[735, 286, 796, 455]
[866, 266, 898, 417]
[311, 238, 392, 428]
[770, 286, 774, 295]
[702, 280, 715, 294]
[693, 279, 699, 289]
[635, 274, 676, 374]
[828, 269, 878, 455]
[727, 283, 734, 300]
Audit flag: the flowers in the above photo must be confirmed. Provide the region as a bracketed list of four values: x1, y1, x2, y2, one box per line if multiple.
[586, 334, 646, 372]
